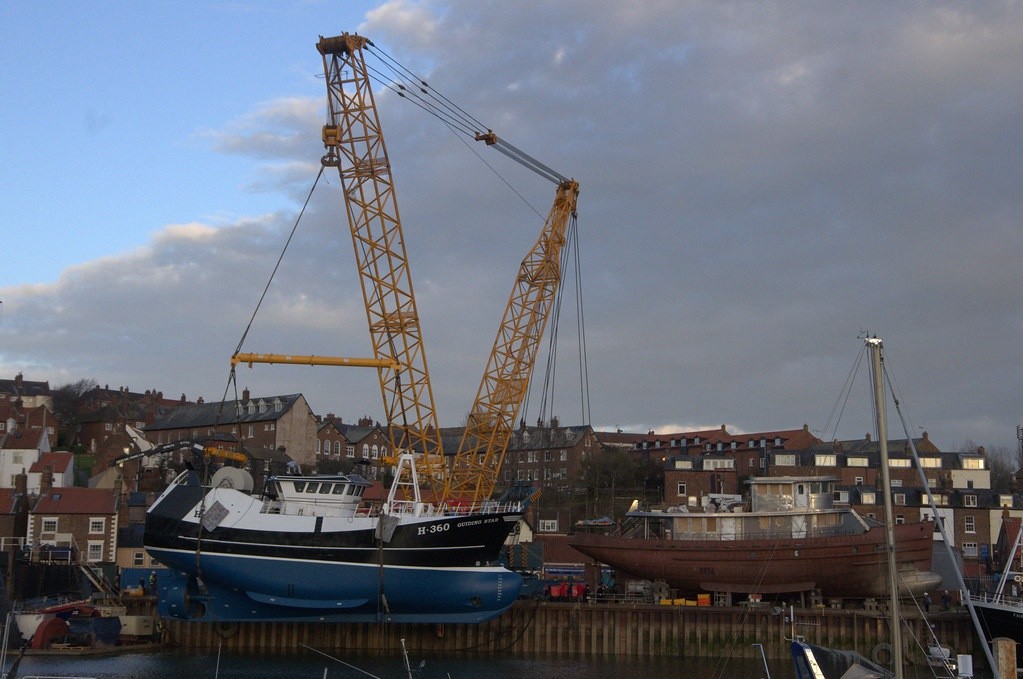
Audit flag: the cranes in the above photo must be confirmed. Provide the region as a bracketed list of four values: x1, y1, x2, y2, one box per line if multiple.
[228, 28, 594, 516]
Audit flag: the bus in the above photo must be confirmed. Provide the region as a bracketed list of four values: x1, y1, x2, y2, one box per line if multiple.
[541, 561, 615, 589]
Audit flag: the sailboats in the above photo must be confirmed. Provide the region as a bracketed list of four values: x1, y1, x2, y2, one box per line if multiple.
[709, 328, 1023, 679]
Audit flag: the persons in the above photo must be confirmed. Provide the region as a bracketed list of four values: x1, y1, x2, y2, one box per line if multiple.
[558, 580, 608, 603]
[941, 590, 951, 609]
[148, 571, 158, 594]
[923, 593, 933, 612]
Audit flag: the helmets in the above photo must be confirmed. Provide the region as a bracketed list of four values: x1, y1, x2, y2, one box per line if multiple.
[152, 570, 156, 574]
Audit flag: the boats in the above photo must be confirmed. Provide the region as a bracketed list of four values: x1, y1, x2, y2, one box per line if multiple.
[564, 515, 945, 601]
[12, 594, 125, 650]
[111, 430, 529, 626]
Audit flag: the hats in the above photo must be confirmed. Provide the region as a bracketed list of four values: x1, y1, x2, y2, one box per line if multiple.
[945, 591, 948, 594]
[924, 592, 928, 595]
